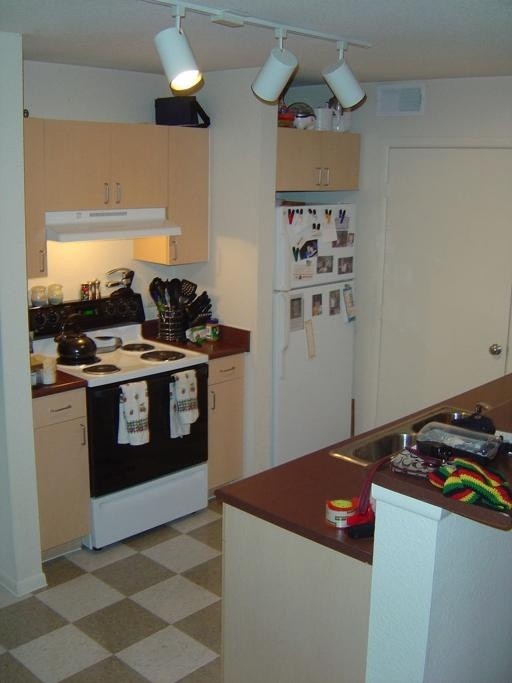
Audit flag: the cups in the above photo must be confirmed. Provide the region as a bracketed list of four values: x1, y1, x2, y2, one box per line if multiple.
[32, 357, 58, 386]
[158, 309, 187, 344]
[315, 107, 353, 133]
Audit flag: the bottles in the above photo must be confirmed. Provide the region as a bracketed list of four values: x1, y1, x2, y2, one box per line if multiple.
[80, 279, 100, 301]
[31, 284, 63, 306]
[205, 318, 220, 342]
[326, 499, 354, 529]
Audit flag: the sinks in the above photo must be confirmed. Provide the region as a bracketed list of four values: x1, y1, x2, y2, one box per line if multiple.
[412, 405, 479, 436]
[327, 429, 418, 471]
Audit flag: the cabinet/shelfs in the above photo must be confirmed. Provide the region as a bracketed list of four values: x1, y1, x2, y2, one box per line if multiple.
[45, 116, 170, 211]
[276, 126, 360, 192]
[32, 381, 91, 560]
[23, 116, 48, 278]
[133, 124, 208, 265]
[206, 354, 250, 493]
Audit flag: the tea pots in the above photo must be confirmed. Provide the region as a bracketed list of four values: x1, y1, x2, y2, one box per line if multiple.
[54, 313, 98, 361]
[292, 109, 316, 130]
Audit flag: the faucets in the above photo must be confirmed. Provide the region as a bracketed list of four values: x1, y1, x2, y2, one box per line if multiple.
[104, 268, 134, 290]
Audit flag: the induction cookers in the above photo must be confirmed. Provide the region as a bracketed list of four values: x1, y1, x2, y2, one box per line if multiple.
[31, 322, 207, 387]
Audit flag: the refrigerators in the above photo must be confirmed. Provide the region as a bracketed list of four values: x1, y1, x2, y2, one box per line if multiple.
[274, 202, 358, 465]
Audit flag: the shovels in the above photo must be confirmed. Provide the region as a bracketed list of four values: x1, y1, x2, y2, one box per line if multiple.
[179, 279, 197, 305]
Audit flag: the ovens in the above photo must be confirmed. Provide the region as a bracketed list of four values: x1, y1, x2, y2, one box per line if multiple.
[88, 365, 209, 549]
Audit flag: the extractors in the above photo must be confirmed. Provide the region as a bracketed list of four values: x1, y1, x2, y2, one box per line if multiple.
[44, 207, 183, 243]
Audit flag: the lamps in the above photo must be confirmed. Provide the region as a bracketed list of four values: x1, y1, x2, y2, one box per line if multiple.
[147, 0, 375, 111]
